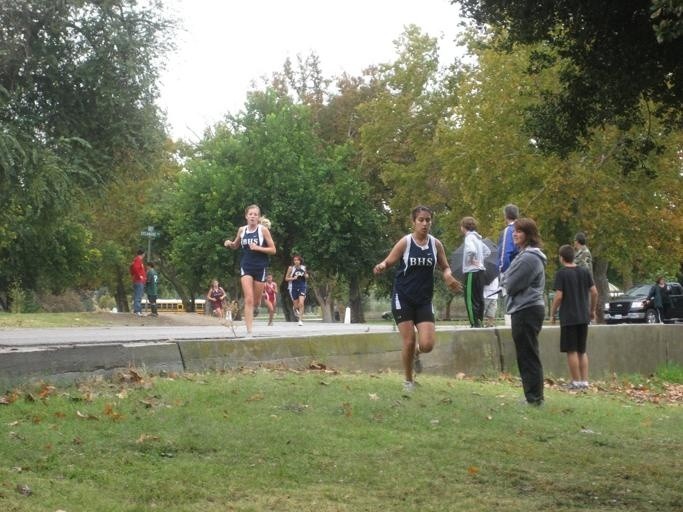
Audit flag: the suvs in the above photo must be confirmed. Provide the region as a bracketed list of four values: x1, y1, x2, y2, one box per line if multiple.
[602, 281, 682, 323]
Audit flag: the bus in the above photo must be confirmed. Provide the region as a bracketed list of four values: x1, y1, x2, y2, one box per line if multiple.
[133, 296, 206, 316]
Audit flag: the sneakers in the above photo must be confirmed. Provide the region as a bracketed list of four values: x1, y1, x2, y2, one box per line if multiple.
[412, 351, 423, 373]
[566, 382, 590, 391]
[401, 381, 415, 398]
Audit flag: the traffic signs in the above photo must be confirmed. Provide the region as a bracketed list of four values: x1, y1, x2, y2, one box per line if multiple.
[139, 230, 162, 238]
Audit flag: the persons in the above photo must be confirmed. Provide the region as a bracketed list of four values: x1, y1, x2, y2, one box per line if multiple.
[572, 233, 593, 325]
[374, 207, 465, 385]
[499, 204, 523, 298]
[502, 216, 547, 404]
[675, 261, 683, 285]
[223, 204, 276, 339]
[460, 216, 492, 327]
[132, 249, 147, 315]
[646, 276, 667, 325]
[551, 244, 599, 388]
[263, 273, 278, 326]
[205, 280, 227, 316]
[145, 262, 159, 316]
[284, 256, 309, 325]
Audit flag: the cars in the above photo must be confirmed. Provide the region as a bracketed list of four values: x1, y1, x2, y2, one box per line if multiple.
[381, 309, 393, 321]
[607, 281, 625, 299]
